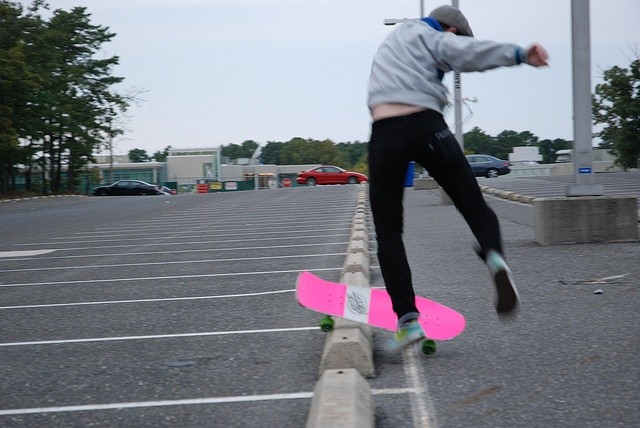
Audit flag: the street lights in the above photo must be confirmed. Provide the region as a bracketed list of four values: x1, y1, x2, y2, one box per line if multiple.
[383, 17, 464, 157]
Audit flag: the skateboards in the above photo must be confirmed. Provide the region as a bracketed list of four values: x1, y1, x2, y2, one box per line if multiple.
[296, 271, 466, 355]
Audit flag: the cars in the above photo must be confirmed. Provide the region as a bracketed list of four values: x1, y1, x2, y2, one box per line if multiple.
[297, 164, 368, 187]
[92, 180, 177, 196]
[464, 154, 512, 178]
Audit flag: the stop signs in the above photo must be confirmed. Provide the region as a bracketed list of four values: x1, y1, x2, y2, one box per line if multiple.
[282, 177, 291, 186]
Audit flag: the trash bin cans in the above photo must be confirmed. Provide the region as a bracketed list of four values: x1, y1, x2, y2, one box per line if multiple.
[258, 173, 274, 190]
[244, 173, 255, 181]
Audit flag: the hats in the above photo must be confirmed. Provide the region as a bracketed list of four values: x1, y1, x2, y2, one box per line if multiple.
[428, 5, 474, 38]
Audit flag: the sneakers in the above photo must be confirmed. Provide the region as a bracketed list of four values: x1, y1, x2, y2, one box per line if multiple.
[384, 322, 425, 353]
[487, 251, 520, 319]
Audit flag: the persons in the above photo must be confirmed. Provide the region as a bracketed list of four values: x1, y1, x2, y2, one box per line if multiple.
[367, 5, 550, 359]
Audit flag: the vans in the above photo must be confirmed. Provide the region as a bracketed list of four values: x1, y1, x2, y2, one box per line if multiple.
[554, 149, 574, 163]
